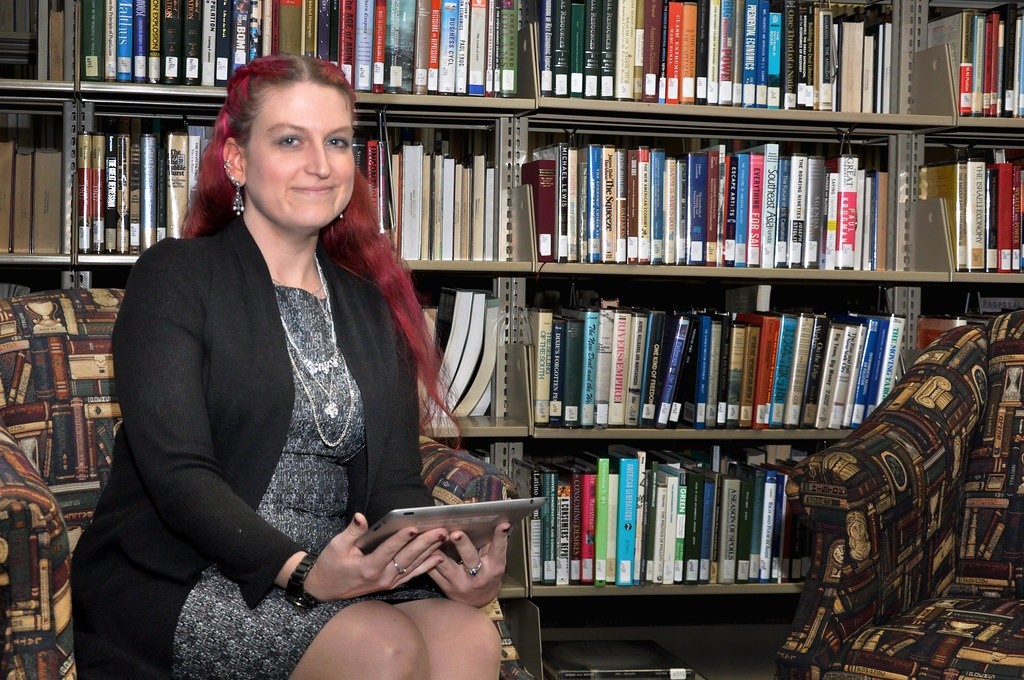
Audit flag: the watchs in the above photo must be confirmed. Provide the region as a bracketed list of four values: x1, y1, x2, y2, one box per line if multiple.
[284, 554, 319, 609]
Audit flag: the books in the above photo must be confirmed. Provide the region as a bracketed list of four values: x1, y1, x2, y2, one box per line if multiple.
[1, 0, 1023, 596]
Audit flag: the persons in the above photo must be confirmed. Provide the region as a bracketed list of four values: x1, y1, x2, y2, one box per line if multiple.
[69, 55, 513, 680]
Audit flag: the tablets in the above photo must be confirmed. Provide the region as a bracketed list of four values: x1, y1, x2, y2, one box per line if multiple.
[355, 497, 550, 562]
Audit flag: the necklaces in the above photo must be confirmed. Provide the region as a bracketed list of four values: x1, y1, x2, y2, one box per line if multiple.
[283, 267, 339, 420]
[280, 250, 341, 373]
[273, 254, 354, 448]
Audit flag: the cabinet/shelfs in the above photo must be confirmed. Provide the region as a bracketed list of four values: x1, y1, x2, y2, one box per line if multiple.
[0, 0, 1024, 599]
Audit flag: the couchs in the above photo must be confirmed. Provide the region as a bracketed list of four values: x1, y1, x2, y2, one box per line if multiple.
[0, 288, 534, 679]
[773, 310, 1024, 680]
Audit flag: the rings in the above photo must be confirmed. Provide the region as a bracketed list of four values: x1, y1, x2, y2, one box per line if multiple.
[463, 561, 483, 575]
[392, 559, 406, 575]
